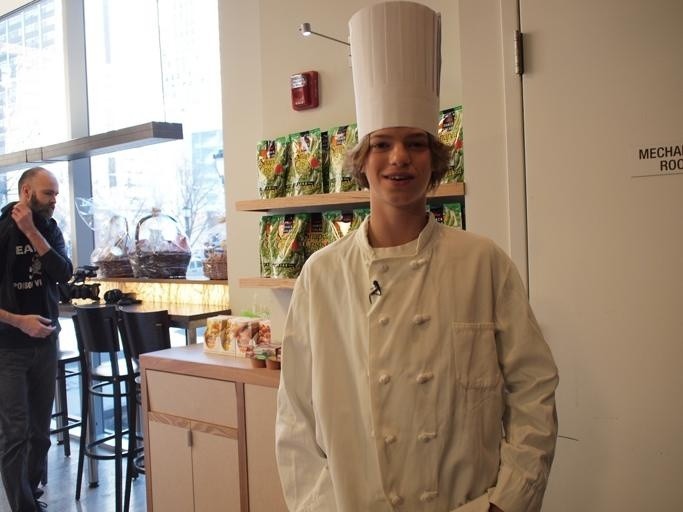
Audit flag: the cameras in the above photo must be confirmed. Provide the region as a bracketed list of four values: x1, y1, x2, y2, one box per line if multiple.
[60, 265, 101, 304]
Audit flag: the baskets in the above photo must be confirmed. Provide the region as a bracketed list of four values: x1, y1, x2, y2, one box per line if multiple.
[128, 213, 192, 279]
[202, 219, 228, 281]
[90, 214, 133, 279]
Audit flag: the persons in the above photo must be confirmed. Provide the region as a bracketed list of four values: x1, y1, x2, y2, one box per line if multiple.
[0, 167, 73, 512]
[273, 1, 559, 512]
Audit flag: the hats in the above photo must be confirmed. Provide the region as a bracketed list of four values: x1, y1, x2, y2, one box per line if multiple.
[348, 1, 442, 142]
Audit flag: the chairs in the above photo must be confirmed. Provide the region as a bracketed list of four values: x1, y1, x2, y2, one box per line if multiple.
[48, 300, 171, 512]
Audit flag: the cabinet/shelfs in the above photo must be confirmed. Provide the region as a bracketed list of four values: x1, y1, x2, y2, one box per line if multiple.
[235, 178, 468, 297]
[144, 367, 246, 512]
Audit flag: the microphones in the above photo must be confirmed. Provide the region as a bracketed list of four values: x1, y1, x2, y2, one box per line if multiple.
[369, 280, 381, 304]
